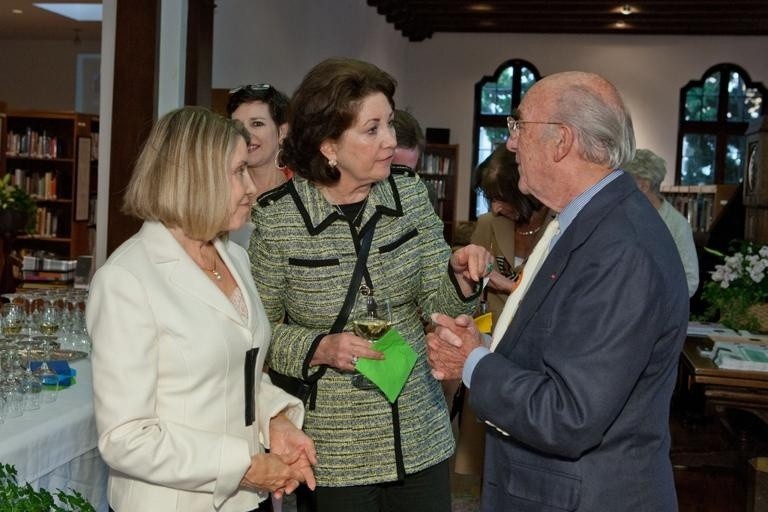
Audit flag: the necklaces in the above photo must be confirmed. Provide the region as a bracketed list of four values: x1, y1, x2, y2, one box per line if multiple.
[516, 226, 541, 236]
[325, 186, 369, 224]
[200, 256, 221, 281]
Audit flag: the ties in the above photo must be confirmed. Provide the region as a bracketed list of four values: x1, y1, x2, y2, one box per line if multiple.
[490, 219, 558, 354]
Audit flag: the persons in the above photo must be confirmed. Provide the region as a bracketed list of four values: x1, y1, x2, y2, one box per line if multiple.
[226, 84, 301, 248]
[454, 144, 556, 477]
[627, 150, 700, 299]
[248, 58, 495, 512]
[392, 109, 435, 209]
[427, 73, 689, 510]
[84, 105, 318, 511]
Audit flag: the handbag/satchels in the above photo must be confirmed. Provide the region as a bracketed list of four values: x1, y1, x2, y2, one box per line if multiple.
[267, 367, 309, 400]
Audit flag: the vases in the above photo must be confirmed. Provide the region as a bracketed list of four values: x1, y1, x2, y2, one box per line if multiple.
[719, 300, 768, 332]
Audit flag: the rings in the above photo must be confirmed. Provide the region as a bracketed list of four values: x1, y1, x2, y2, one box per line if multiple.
[351, 357, 358, 366]
[487, 263, 494, 272]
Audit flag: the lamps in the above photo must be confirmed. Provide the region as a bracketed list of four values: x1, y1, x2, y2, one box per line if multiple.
[621, 0, 631, 14]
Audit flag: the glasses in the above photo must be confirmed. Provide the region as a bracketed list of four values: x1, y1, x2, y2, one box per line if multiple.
[228, 83, 269, 95]
[506, 115, 563, 139]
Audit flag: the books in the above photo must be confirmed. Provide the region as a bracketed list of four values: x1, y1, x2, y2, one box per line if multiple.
[664, 195, 712, 234]
[687, 321, 768, 373]
[417, 153, 450, 217]
[6, 125, 98, 292]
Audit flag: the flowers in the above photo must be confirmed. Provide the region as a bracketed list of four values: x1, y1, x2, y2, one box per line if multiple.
[699, 247, 768, 333]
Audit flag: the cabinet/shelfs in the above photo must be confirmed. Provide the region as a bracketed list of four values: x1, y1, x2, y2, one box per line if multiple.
[0, 108, 99, 281]
[417, 141, 459, 231]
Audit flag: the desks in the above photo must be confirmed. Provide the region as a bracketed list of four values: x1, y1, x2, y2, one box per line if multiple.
[668, 316, 768, 510]
[1, 327, 110, 512]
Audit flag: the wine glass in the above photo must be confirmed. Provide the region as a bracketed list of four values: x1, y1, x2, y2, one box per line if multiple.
[0, 289, 91, 418]
[352, 290, 391, 390]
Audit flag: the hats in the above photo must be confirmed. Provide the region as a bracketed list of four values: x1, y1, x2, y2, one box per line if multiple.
[627, 151, 664, 180]
